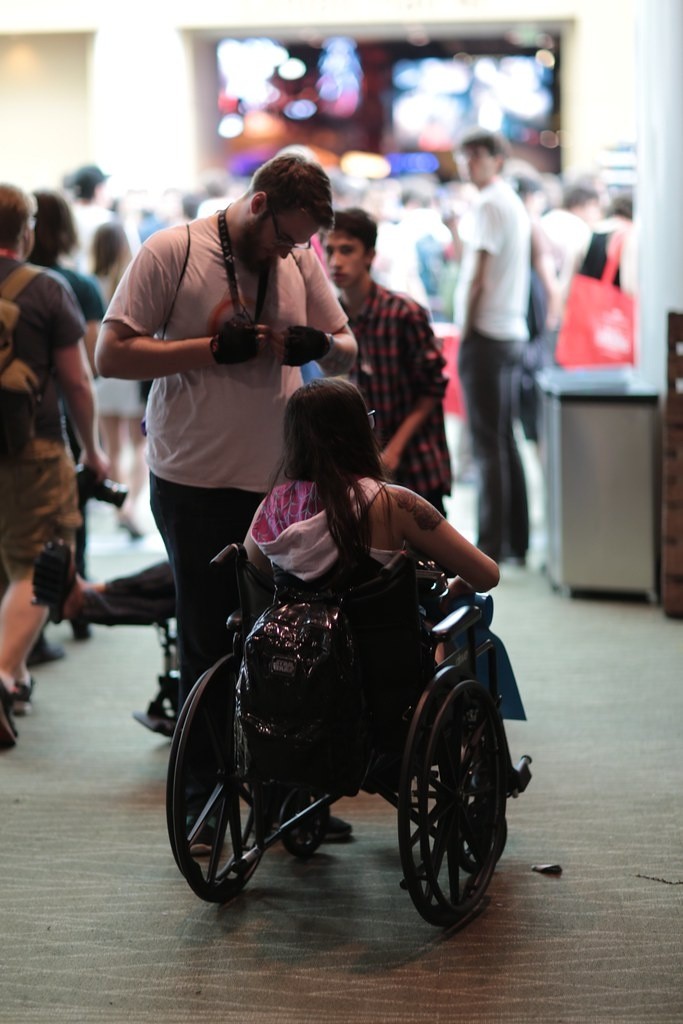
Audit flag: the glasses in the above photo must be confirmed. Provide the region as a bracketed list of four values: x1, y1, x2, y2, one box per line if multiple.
[366, 409, 376, 430]
[268, 205, 311, 250]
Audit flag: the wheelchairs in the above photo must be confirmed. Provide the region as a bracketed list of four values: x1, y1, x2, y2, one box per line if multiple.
[153, 544, 544, 935]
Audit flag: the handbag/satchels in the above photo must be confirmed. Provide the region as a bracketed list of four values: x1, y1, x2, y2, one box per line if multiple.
[554, 229, 634, 366]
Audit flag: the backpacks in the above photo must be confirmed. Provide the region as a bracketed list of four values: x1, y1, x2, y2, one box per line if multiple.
[237, 561, 373, 796]
[0, 262, 45, 458]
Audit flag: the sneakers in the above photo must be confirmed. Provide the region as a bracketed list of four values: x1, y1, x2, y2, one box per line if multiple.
[0, 673, 34, 746]
[250, 810, 352, 841]
[185, 822, 215, 853]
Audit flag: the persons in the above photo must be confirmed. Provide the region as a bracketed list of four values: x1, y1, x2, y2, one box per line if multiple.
[10, 125, 637, 667]
[93, 152, 356, 858]
[238, 377, 519, 795]
[0, 183, 109, 745]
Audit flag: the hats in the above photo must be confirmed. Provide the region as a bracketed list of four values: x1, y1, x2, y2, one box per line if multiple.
[74, 166, 112, 187]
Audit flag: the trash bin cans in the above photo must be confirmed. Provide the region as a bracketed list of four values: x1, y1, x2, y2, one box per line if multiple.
[536, 367, 664, 604]
